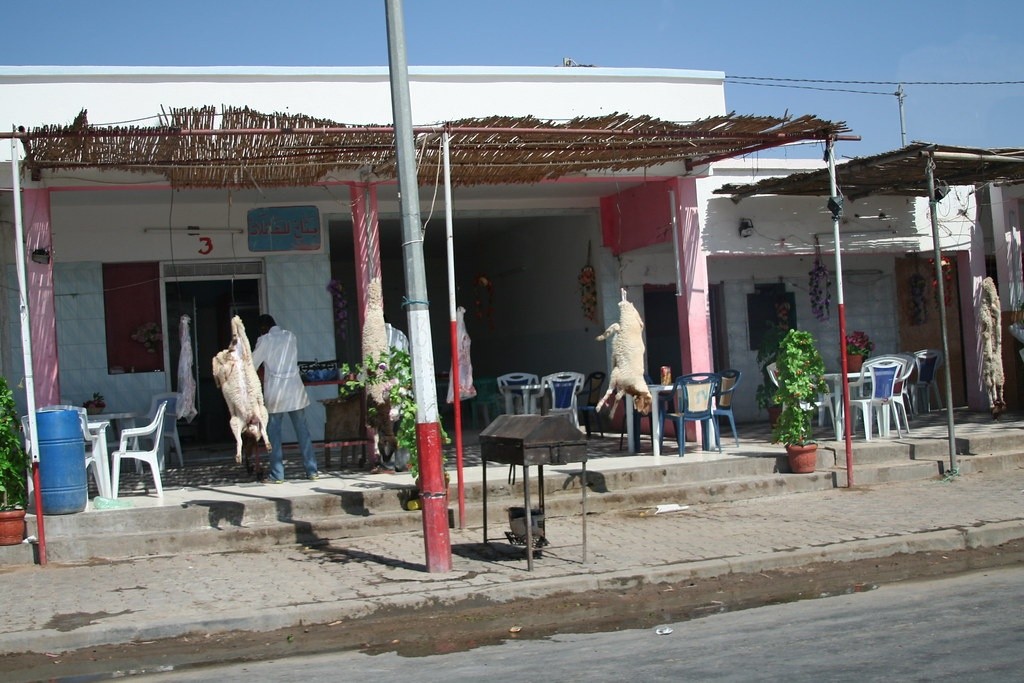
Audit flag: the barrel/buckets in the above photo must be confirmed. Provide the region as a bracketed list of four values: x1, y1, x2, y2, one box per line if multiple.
[36, 409, 88, 515]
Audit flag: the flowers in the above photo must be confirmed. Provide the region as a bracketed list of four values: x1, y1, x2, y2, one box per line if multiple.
[909, 272, 929, 326]
[334, 343, 452, 483]
[929, 252, 953, 311]
[327, 279, 349, 340]
[769, 329, 829, 449]
[472, 271, 497, 334]
[129, 321, 163, 355]
[579, 267, 599, 326]
[807, 260, 832, 322]
[774, 294, 791, 329]
[82, 392, 105, 408]
[839, 329, 876, 357]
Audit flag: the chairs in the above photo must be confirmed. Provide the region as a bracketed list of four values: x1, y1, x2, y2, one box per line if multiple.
[766, 349, 944, 442]
[471, 371, 607, 441]
[20, 391, 183, 514]
[614, 368, 741, 458]
[317, 383, 396, 468]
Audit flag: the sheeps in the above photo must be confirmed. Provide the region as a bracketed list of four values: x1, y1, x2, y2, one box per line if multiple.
[212, 313, 273, 463]
[595, 301, 654, 420]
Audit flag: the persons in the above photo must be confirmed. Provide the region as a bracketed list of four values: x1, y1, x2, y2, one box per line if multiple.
[253, 314, 319, 484]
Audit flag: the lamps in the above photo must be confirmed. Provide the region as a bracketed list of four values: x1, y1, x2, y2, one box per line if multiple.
[827, 196, 844, 219]
[144, 229, 244, 234]
[32, 249, 50, 265]
[935, 180, 951, 200]
[739, 222, 753, 237]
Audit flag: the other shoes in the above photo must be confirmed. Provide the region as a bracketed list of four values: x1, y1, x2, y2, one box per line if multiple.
[308, 474, 320, 481]
[264, 478, 282, 483]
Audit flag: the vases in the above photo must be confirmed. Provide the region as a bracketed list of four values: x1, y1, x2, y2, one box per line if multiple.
[845, 355, 863, 373]
[785, 443, 818, 473]
[86, 408, 104, 415]
[414, 478, 450, 508]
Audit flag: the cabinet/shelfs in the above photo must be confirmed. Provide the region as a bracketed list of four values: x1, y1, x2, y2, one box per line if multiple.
[242, 368, 381, 482]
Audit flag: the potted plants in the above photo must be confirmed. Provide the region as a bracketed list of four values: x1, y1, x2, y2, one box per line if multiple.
[755, 320, 790, 429]
[0, 376, 33, 545]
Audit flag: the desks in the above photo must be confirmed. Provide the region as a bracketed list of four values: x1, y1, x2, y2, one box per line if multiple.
[809, 372, 898, 442]
[626, 382, 717, 457]
[502, 383, 581, 417]
[87, 411, 144, 474]
